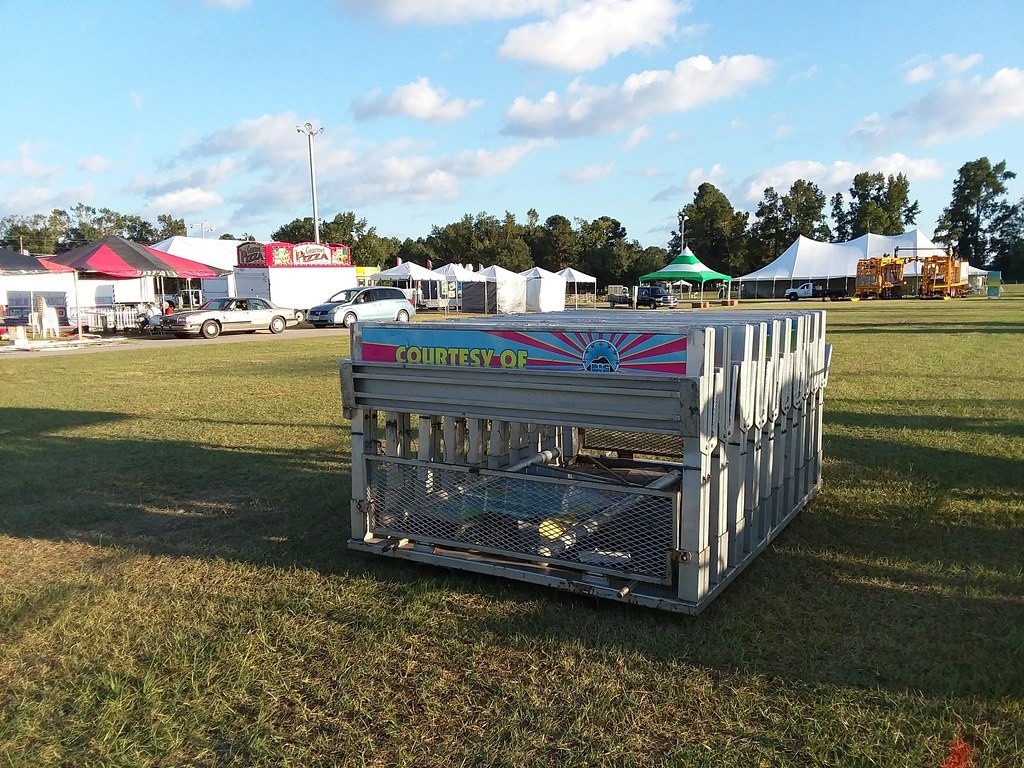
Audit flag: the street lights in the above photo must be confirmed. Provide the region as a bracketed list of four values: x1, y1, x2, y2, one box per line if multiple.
[296, 122, 326, 243]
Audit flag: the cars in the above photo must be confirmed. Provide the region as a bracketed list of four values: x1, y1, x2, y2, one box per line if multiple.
[305, 286, 417, 329]
[165, 290, 199, 310]
[162, 297, 299, 340]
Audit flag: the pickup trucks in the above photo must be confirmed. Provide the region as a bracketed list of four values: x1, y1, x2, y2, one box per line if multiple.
[785, 282, 846, 301]
[608, 286, 678, 309]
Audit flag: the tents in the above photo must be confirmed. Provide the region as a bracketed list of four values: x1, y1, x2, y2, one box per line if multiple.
[429, 262, 487, 315]
[638, 245, 731, 307]
[554, 267, 596, 310]
[518, 266, 566, 313]
[372, 261, 447, 316]
[45, 235, 233, 341]
[475, 265, 527, 315]
[0, 247, 82, 341]
[672, 279, 692, 299]
[726, 230, 998, 299]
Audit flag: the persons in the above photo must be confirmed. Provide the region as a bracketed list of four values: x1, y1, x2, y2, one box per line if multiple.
[138, 302, 173, 334]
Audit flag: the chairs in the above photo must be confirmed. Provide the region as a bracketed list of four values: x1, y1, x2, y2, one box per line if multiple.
[26, 296, 59, 338]
